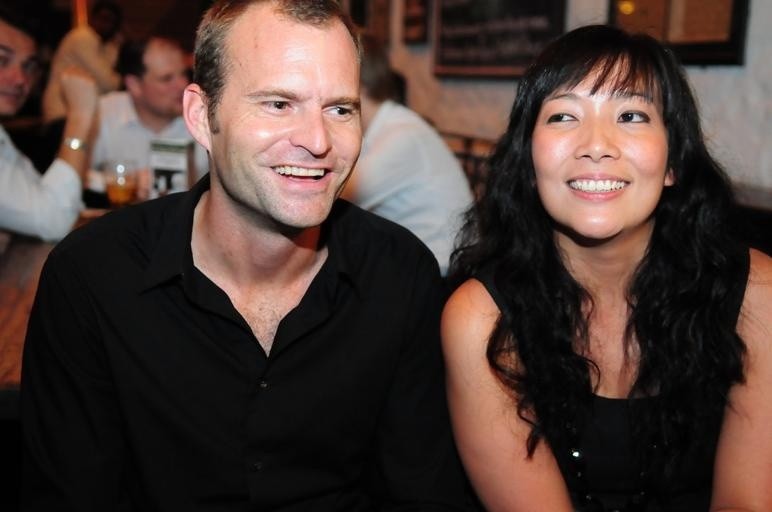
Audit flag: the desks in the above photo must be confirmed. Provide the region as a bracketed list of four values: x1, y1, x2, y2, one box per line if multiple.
[0, 207, 146, 394]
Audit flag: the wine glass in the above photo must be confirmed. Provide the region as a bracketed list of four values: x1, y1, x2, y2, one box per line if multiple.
[106, 158, 137, 213]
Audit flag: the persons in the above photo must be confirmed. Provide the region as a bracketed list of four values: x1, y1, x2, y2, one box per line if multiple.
[20, 0, 487, 512]
[332, 27, 479, 274]
[1, 2, 211, 275]
[439, 24, 772, 512]
